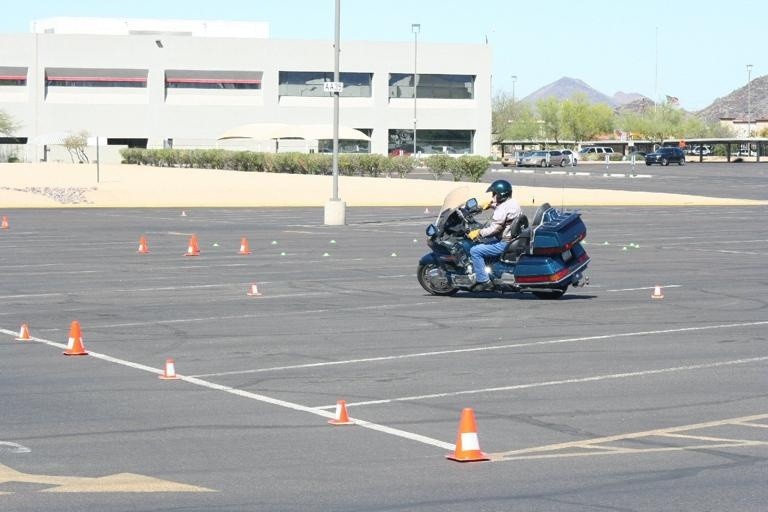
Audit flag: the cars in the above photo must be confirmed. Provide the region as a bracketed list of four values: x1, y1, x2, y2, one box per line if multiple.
[643, 147, 685, 167]
[550, 150, 580, 166]
[522, 149, 570, 168]
[318, 148, 331, 153]
[347, 146, 368, 153]
[501, 150, 539, 167]
[628, 151, 647, 157]
[420, 145, 458, 153]
[732, 147, 757, 157]
[388, 145, 426, 158]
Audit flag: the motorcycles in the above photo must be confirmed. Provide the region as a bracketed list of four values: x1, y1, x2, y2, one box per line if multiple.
[416, 187, 591, 299]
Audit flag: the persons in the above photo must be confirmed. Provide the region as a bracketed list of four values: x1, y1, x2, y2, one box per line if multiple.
[468, 179, 522, 292]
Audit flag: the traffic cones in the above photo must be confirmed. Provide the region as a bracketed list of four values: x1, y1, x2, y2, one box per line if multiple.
[326, 398, 357, 427]
[62, 319, 88, 356]
[15, 323, 33, 341]
[650, 283, 665, 299]
[246, 283, 263, 298]
[158, 358, 180, 381]
[1, 216, 10, 230]
[444, 405, 491, 463]
[182, 233, 200, 257]
[136, 236, 151, 253]
[236, 237, 252, 256]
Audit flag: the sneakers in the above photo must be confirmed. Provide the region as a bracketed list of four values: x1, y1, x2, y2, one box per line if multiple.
[473, 280, 494, 292]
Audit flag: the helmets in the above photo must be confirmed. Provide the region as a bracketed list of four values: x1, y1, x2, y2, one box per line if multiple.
[485, 180, 512, 203]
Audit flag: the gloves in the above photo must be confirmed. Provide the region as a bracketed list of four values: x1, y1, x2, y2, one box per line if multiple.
[468, 229, 481, 240]
[479, 201, 493, 209]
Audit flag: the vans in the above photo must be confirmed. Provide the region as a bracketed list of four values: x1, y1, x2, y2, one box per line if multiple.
[577, 146, 615, 155]
[683, 145, 711, 156]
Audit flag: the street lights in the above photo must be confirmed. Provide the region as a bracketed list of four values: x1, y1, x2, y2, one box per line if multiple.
[746, 64, 752, 157]
[510, 75, 517, 141]
[411, 23, 421, 156]
